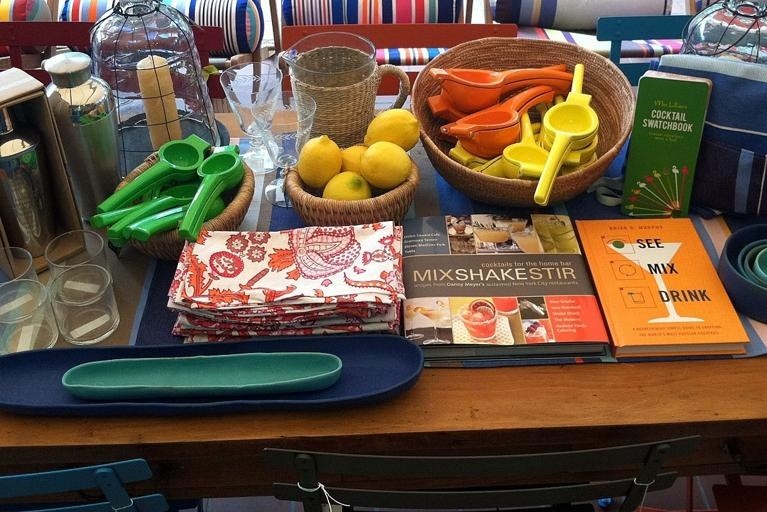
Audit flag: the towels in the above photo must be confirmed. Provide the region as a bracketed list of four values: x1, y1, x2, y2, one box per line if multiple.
[165, 220, 408, 345]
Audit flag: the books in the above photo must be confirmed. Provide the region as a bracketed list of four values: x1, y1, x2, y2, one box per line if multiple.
[570, 216, 750, 359]
[400, 210, 611, 362]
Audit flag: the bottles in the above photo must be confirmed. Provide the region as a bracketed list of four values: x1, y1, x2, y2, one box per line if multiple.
[44, 49, 124, 227]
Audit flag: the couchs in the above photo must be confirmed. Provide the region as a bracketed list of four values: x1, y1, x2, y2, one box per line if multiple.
[0, 0, 767, 96]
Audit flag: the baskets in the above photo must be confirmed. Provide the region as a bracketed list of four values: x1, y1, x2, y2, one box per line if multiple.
[410, 35, 639, 211]
[109, 141, 254, 260]
[288, 146, 418, 232]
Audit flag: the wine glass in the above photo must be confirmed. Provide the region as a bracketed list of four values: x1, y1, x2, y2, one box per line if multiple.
[420, 305, 451, 346]
[253, 87, 317, 207]
[405, 307, 424, 342]
[219, 61, 284, 176]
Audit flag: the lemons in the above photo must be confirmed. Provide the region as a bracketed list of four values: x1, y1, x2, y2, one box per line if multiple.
[298, 109, 421, 201]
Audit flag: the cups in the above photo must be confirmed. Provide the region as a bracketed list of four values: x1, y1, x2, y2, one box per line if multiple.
[0, 139, 58, 258]
[276, 29, 412, 159]
[459, 304, 497, 340]
[450, 218, 544, 254]
[45, 230, 116, 285]
[0, 245, 39, 288]
[50, 264, 120, 345]
[0, 279, 58, 356]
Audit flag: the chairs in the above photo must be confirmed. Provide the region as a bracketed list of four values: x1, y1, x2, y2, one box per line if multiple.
[262, 431, 702, 510]
[0, 457, 203, 510]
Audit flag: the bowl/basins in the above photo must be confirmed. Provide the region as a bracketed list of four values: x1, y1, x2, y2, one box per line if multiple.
[717, 221, 767, 322]
